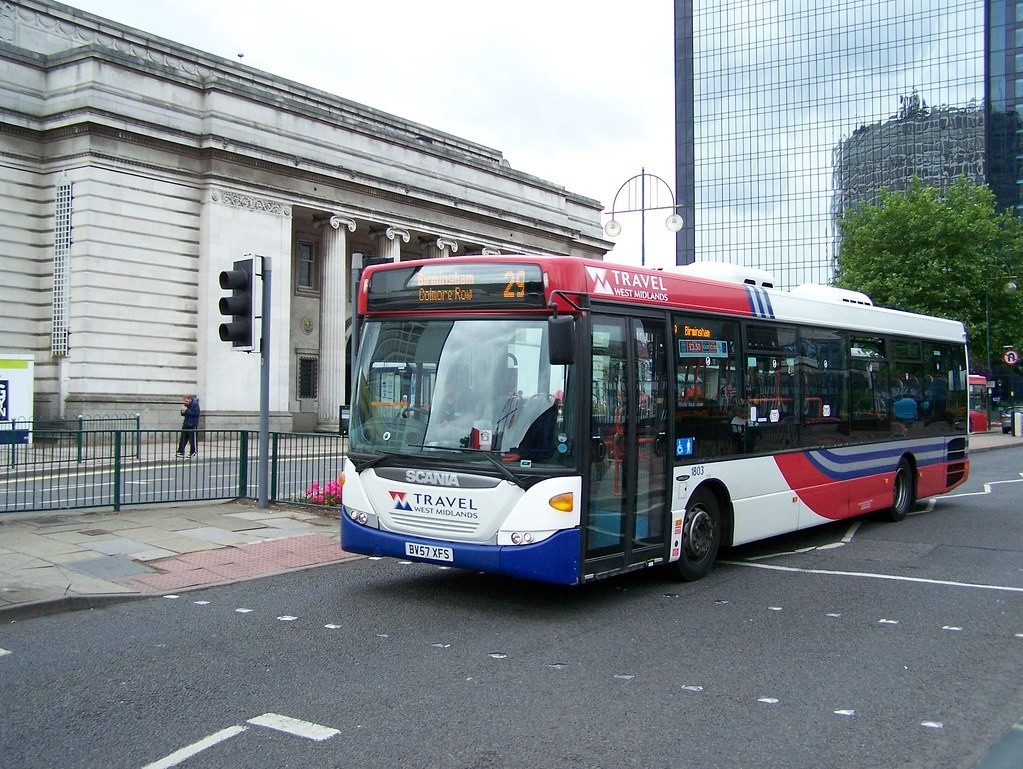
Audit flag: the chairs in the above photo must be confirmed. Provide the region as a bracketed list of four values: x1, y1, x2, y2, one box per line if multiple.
[503, 393, 559, 460]
[859, 374, 949, 428]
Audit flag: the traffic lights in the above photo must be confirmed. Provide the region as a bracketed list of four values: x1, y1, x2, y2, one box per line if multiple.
[219, 257, 253, 348]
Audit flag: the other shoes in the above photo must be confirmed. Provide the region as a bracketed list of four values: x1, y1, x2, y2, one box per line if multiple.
[176, 453, 185, 457]
[186, 453, 195, 457]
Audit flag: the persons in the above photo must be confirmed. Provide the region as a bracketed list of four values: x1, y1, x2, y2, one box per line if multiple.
[395, 394, 413, 419]
[175, 395, 200, 458]
[426, 364, 477, 430]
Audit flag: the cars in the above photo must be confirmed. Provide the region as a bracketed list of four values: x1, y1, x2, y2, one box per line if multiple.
[1001, 405, 1023, 434]
[654, 396, 733, 456]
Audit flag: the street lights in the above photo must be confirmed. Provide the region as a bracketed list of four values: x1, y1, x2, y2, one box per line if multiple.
[603, 167, 684, 265]
[983, 253, 1019, 431]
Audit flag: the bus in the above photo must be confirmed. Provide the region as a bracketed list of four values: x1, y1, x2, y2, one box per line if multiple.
[332, 256, 973, 590]
[746, 396, 823, 428]
[969, 373, 988, 434]
